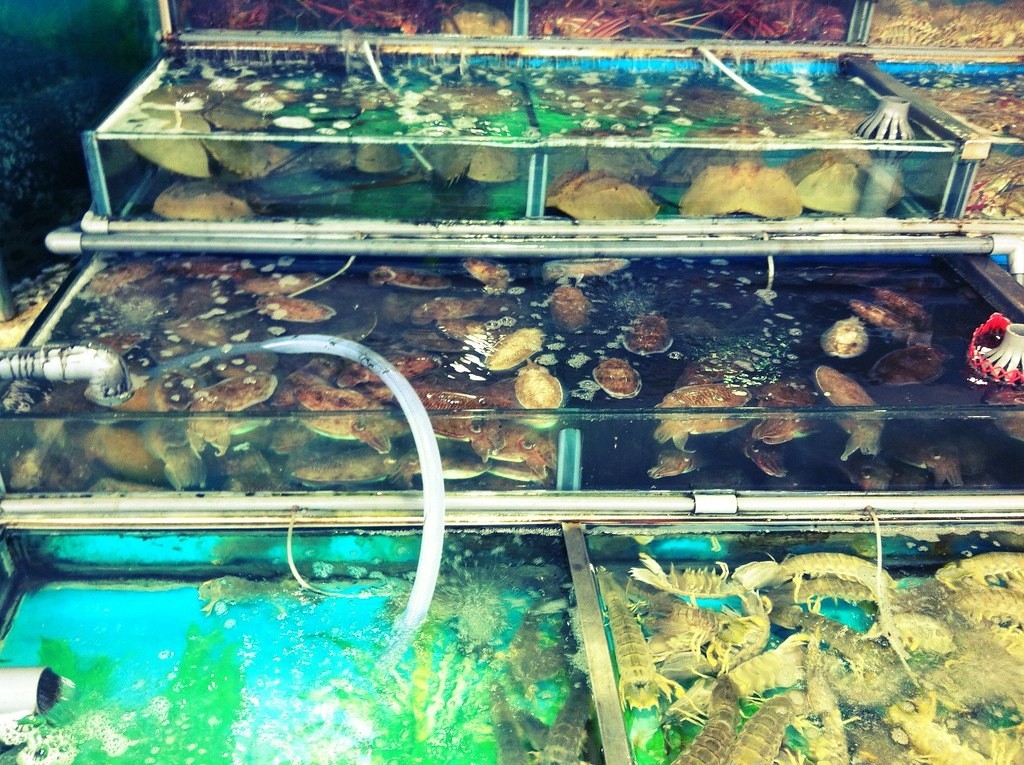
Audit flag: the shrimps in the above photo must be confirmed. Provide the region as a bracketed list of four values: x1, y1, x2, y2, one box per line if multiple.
[198, 567, 324, 608]
[380, 551, 1023, 765]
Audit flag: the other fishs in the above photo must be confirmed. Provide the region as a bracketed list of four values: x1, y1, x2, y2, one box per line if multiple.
[20, 244, 1023, 492]
